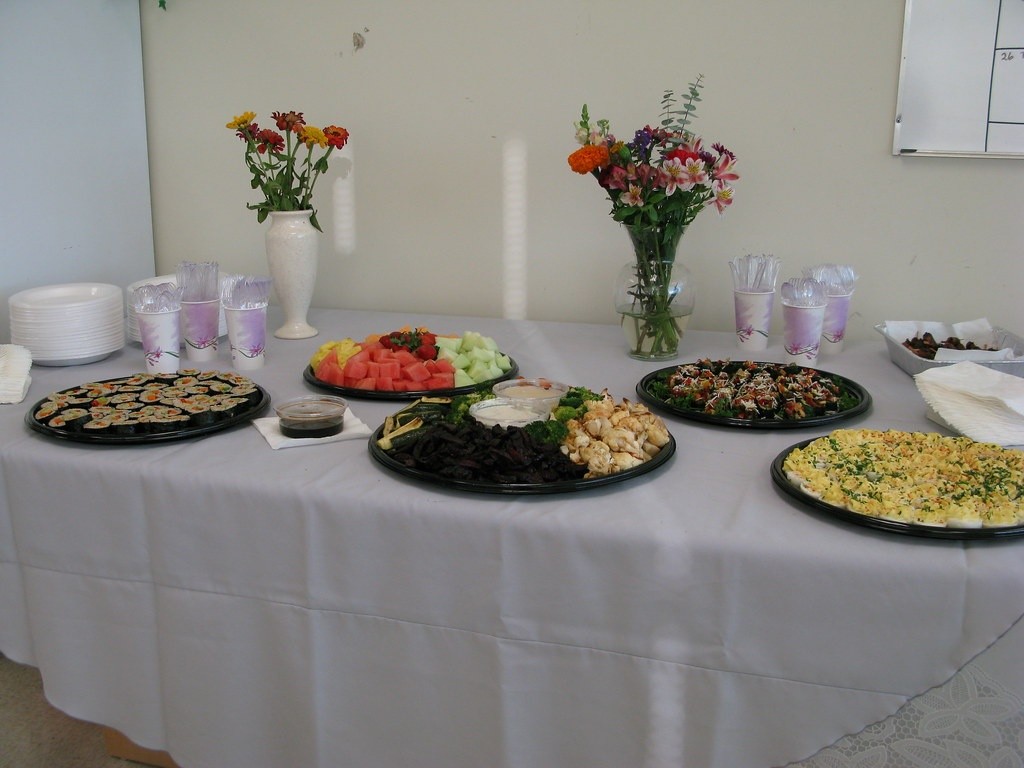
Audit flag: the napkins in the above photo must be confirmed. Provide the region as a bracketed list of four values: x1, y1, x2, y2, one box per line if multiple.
[913, 360, 1023, 448]
[0, 344, 33, 404]
[251, 406, 373, 450]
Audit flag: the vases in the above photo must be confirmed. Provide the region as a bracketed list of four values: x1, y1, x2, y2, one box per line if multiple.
[265, 210, 319, 339]
[614, 224, 694, 362]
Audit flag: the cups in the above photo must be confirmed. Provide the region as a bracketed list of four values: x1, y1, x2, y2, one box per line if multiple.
[224, 303, 269, 370]
[733, 288, 775, 352]
[134, 306, 182, 374]
[782, 302, 827, 368]
[821, 294, 851, 357]
[179, 299, 221, 361]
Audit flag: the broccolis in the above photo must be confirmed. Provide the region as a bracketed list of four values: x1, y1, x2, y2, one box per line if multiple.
[443, 384, 604, 444]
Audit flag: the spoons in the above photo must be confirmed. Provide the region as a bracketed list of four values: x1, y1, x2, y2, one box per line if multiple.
[131, 260, 274, 311]
[730, 252, 855, 306]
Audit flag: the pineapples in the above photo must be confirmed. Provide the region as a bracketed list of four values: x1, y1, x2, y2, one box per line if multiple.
[311, 337, 362, 374]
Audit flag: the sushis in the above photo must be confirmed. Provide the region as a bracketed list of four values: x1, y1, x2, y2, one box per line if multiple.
[34, 368, 261, 436]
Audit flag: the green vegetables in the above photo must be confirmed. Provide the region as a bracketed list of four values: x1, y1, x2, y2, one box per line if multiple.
[651, 361, 862, 420]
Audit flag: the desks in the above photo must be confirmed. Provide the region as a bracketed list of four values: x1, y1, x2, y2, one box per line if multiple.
[0, 307, 1024, 768]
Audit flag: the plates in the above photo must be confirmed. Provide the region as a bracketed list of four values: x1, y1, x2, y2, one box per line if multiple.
[24, 376, 271, 445]
[7, 271, 231, 367]
[636, 361, 873, 429]
[770, 434, 1024, 540]
[367, 390, 677, 493]
[302, 352, 520, 399]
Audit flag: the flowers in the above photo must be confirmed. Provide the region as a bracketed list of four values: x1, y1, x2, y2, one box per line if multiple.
[226, 110, 349, 233]
[568, 72, 740, 356]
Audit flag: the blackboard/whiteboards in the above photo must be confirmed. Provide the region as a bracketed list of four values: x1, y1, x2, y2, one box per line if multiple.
[892, 0, 1024, 161]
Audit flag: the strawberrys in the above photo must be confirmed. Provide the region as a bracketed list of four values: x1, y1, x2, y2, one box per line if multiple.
[379, 331, 439, 361]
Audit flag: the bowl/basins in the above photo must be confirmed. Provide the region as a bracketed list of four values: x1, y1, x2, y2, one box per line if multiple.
[492, 378, 570, 409]
[872, 322, 1024, 377]
[468, 398, 551, 429]
[273, 394, 348, 438]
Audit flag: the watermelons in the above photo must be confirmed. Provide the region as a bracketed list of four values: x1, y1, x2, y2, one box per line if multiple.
[317, 341, 454, 392]
